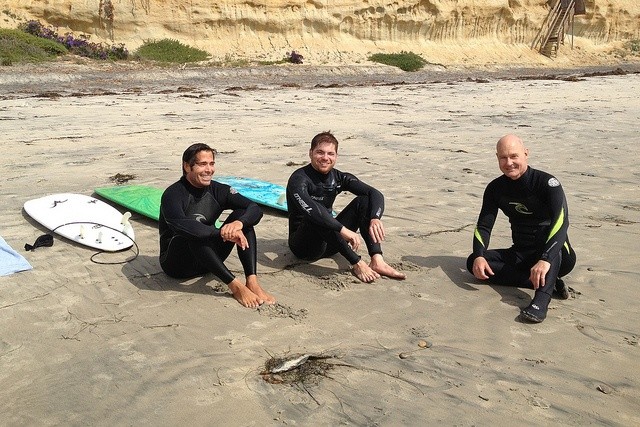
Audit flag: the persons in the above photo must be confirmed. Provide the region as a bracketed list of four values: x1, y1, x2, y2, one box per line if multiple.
[286, 132, 407, 283]
[467, 133, 576, 323]
[159, 143, 276, 309]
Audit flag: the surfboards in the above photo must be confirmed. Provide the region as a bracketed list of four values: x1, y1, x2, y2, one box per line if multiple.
[94, 186, 164, 222]
[211, 177, 288, 213]
[24, 192, 136, 253]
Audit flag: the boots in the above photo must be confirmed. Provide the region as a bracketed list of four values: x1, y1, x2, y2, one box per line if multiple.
[530, 270, 569, 299]
[520, 272, 557, 323]
